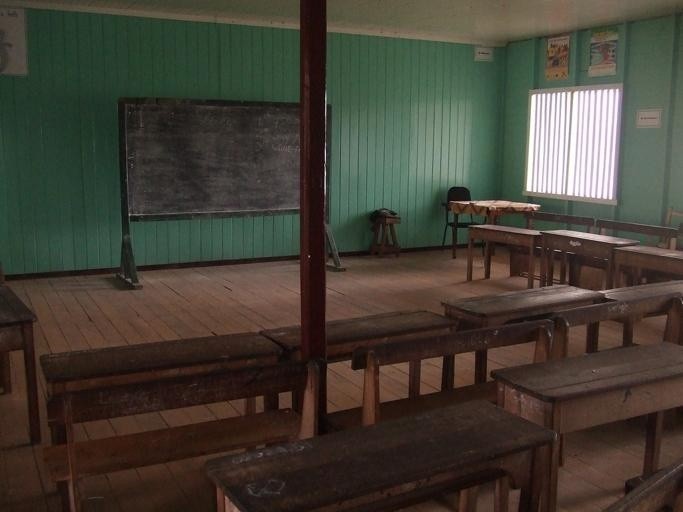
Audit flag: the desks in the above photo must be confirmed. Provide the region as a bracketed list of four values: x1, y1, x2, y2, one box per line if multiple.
[0, 201, 683, 510]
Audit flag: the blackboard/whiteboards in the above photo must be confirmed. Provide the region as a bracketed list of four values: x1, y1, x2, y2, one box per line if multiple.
[117, 96, 332, 222]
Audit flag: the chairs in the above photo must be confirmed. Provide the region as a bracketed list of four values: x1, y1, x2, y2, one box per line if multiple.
[442, 184, 487, 259]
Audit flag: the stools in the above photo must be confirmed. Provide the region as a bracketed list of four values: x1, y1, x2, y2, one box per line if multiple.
[368, 211, 401, 257]
